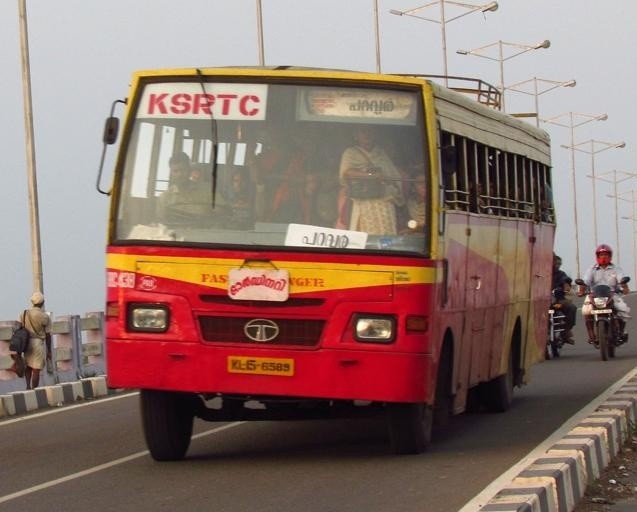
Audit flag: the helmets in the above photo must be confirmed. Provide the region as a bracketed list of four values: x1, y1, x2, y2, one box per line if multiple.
[595, 245, 611, 265]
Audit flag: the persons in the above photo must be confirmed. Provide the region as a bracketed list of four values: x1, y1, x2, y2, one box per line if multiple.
[549, 251, 577, 344]
[230, 119, 549, 235]
[154, 152, 229, 222]
[19, 291, 51, 390]
[576, 244, 629, 343]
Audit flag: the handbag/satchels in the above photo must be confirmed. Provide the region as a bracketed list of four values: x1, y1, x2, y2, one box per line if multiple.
[346, 145, 386, 199]
[9, 309, 29, 355]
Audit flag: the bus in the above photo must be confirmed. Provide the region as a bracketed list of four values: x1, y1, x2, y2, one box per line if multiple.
[95, 64, 555, 460]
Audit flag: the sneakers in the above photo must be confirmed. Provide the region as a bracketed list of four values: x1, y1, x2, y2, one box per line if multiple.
[563, 338, 575, 344]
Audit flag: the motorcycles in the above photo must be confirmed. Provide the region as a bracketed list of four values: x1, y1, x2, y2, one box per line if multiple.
[574, 276, 629, 359]
[547, 276, 572, 357]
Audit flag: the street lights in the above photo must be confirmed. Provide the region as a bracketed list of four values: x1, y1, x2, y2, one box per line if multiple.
[560, 139, 625, 249]
[389, 0, 498, 91]
[585, 170, 636, 276]
[541, 113, 614, 292]
[455, 40, 550, 113]
[494, 77, 576, 129]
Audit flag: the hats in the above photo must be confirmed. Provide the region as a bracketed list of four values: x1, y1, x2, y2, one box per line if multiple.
[30, 292, 44, 305]
